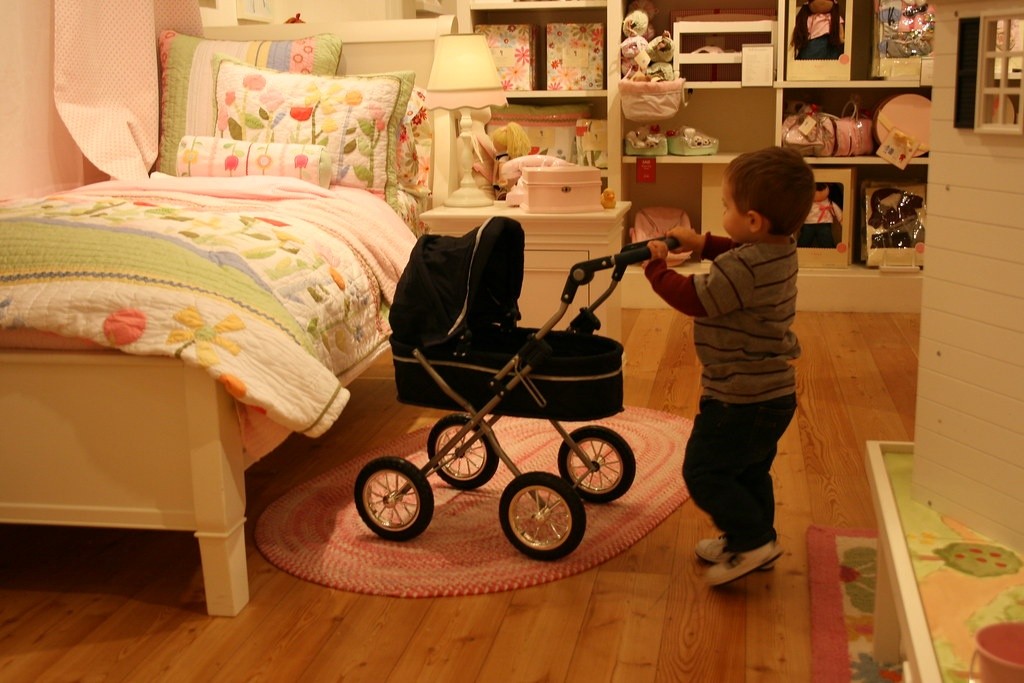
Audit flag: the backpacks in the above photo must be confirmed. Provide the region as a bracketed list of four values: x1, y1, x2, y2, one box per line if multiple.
[630, 206, 695, 268]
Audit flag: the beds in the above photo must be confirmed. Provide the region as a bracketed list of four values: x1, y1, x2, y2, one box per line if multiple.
[0, 14, 458, 616]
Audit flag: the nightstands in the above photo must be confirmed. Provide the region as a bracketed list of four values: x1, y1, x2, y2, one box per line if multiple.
[418, 200, 632, 342]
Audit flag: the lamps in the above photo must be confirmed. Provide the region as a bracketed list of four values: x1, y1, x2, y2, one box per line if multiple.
[422, 33, 509, 208]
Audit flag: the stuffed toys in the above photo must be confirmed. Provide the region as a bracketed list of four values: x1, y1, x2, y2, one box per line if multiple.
[629, 125, 712, 149]
[791, 0, 846, 60]
[476, 123, 530, 199]
[798, 183, 843, 247]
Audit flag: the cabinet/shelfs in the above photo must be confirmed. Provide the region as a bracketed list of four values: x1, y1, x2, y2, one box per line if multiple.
[673, 14, 777, 79]
[456, 0, 935, 313]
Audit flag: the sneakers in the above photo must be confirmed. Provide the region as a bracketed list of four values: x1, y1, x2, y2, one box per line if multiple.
[694, 532, 774, 569]
[704, 538, 784, 586]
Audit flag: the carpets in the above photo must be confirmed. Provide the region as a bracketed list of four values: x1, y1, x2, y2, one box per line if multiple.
[255, 405, 694, 599]
[807, 524, 905, 683]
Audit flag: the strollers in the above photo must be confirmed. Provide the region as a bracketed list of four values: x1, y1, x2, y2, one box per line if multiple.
[354, 216, 681, 561]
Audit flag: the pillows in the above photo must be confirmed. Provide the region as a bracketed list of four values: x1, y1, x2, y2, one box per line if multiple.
[211, 54, 416, 211]
[175, 136, 331, 188]
[397, 86, 432, 199]
[154, 28, 343, 175]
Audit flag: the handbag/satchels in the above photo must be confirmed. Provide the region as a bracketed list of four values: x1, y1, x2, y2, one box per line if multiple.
[781, 101, 838, 157]
[817, 100, 875, 157]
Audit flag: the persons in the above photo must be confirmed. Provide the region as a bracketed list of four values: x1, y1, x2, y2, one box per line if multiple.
[643, 146, 815, 587]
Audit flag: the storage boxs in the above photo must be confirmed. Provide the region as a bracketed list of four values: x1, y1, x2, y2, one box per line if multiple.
[546, 22, 604, 91]
[475, 22, 539, 91]
[786, 0, 853, 81]
[575, 118, 608, 168]
[701, 164, 855, 270]
[486, 102, 591, 162]
[520, 166, 604, 213]
